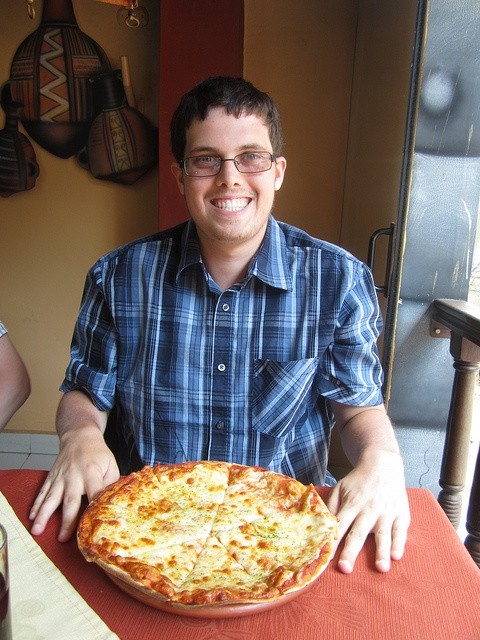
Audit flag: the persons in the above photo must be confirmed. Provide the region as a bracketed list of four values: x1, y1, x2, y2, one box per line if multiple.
[26, 75, 413, 577]
[1, 321, 34, 432]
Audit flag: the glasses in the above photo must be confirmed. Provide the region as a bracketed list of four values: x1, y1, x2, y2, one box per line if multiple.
[180, 151, 273, 176]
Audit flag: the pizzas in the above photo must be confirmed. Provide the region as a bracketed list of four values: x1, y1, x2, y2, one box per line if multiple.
[76, 461, 340, 609]
